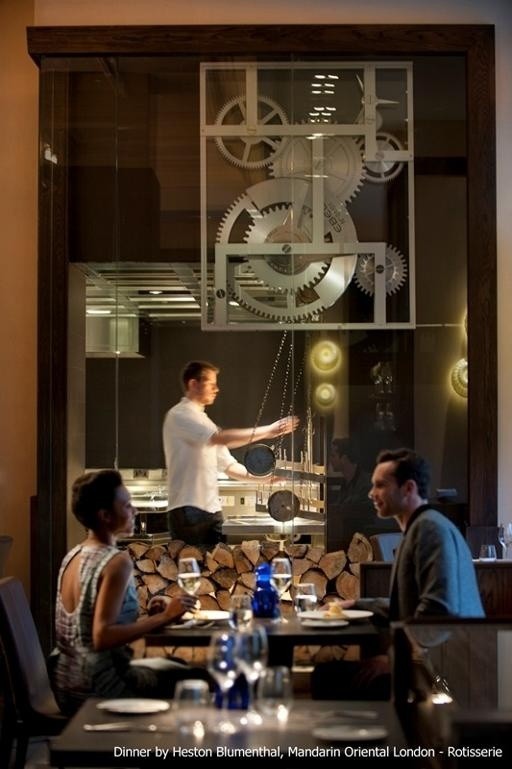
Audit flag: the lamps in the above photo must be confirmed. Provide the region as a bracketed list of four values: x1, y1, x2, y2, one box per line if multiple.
[419, 655, 456, 706]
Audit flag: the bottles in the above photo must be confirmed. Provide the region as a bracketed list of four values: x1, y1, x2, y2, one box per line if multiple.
[247, 560, 279, 618]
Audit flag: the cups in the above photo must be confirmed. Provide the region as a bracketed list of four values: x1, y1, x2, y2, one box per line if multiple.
[479, 543, 498, 561]
[171, 679, 210, 731]
[257, 666, 294, 716]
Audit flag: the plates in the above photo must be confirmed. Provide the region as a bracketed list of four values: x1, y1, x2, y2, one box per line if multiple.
[192, 608, 232, 621]
[313, 723, 389, 744]
[297, 608, 374, 621]
[97, 696, 169, 715]
[303, 621, 347, 629]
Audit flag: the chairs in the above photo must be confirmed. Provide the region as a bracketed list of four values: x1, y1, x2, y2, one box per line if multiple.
[368, 523, 505, 563]
[1, 573, 70, 769]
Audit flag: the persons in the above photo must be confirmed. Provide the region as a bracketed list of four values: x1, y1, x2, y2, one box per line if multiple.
[311, 448, 487, 701]
[55, 470, 215, 701]
[163, 360, 299, 555]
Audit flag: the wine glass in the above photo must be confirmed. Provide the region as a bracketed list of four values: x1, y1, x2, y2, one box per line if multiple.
[498, 522, 512, 561]
[176, 558, 203, 626]
[210, 625, 267, 738]
[268, 556, 318, 626]
[229, 593, 254, 632]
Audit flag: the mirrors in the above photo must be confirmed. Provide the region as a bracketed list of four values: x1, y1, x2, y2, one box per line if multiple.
[84, 282, 353, 518]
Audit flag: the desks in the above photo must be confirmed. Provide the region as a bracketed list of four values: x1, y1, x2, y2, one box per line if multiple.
[50, 602, 408, 767]
